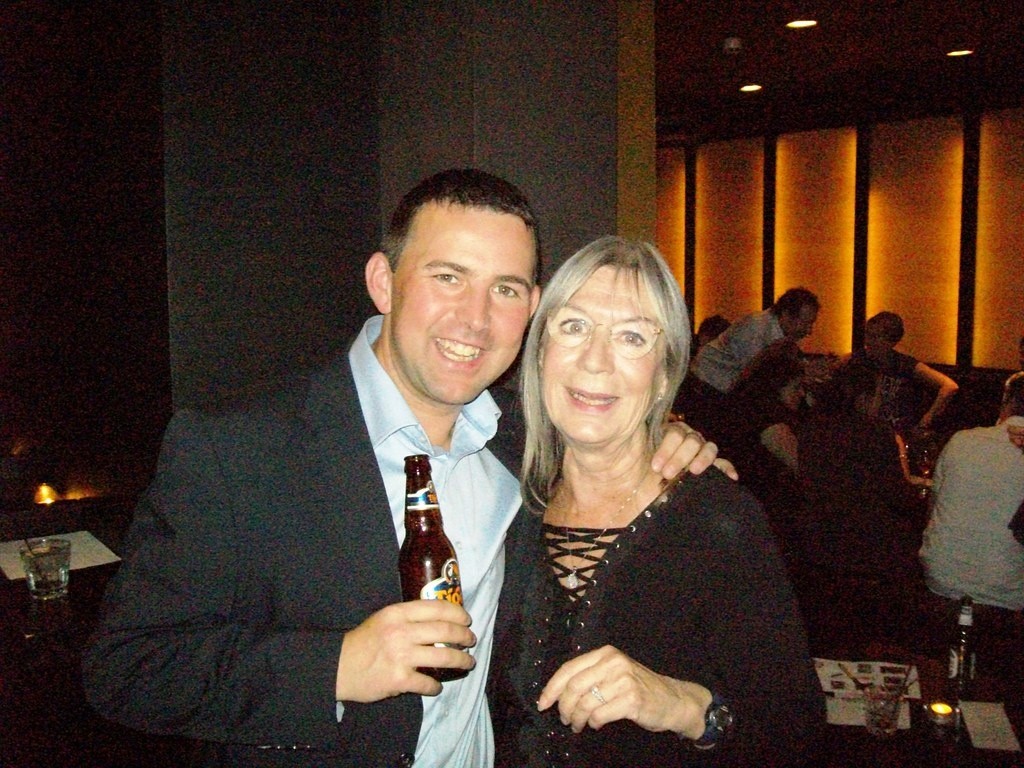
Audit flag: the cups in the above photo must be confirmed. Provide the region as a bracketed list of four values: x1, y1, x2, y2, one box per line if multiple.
[861, 683, 907, 738]
[21, 539, 73, 600]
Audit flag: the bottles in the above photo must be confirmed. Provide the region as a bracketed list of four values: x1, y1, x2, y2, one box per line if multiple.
[399, 454, 470, 683]
[948, 594, 978, 700]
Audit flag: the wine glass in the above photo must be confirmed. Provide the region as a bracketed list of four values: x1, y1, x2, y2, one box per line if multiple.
[916, 445, 935, 496]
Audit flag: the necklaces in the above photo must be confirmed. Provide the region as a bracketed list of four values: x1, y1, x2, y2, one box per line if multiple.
[559, 478, 637, 589]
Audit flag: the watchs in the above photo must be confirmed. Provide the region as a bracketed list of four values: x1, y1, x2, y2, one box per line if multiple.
[692, 692, 733, 747]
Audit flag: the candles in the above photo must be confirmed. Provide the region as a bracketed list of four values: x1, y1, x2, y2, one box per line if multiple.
[929, 702, 953, 725]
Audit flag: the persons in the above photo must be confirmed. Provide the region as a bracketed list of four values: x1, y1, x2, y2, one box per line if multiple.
[918, 371, 1024, 610]
[80, 167, 738, 768]
[689, 288, 959, 499]
[484, 234, 828, 768]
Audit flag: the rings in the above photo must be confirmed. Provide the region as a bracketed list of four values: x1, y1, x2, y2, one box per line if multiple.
[591, 685, 608, 704]
[664, 423, 706, 446]
